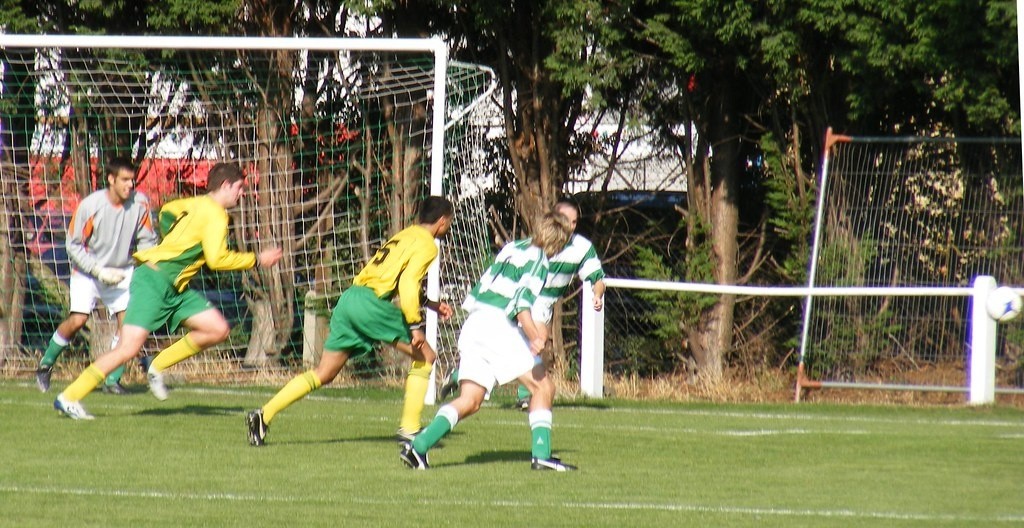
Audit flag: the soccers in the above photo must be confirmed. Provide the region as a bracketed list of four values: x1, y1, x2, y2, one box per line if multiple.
[988, 286, 1022, 323]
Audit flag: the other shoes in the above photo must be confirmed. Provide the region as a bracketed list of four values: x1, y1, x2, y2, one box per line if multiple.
[516, 399, 530, 411]
[437, 367, 458, 403]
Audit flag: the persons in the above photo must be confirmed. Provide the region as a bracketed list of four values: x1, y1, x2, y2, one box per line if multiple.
[245, 197, 453, 441]
[54, 163, 283, 419]
[442, 200, 605, 410]
[35, 157, 157, 395]
[401, 213, 579, 474]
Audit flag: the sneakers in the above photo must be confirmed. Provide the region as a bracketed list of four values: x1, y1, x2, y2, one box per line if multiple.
[245, 408, 270, 448]
[102, 378, 132, 396]
[395, 427, 445, 449]
[138, 356, 168, 401]
[530, 457, 578, 473]
[399, 442, 430, 470]
[36, 357, 54, 393]
[53, 393, 95, 421]
